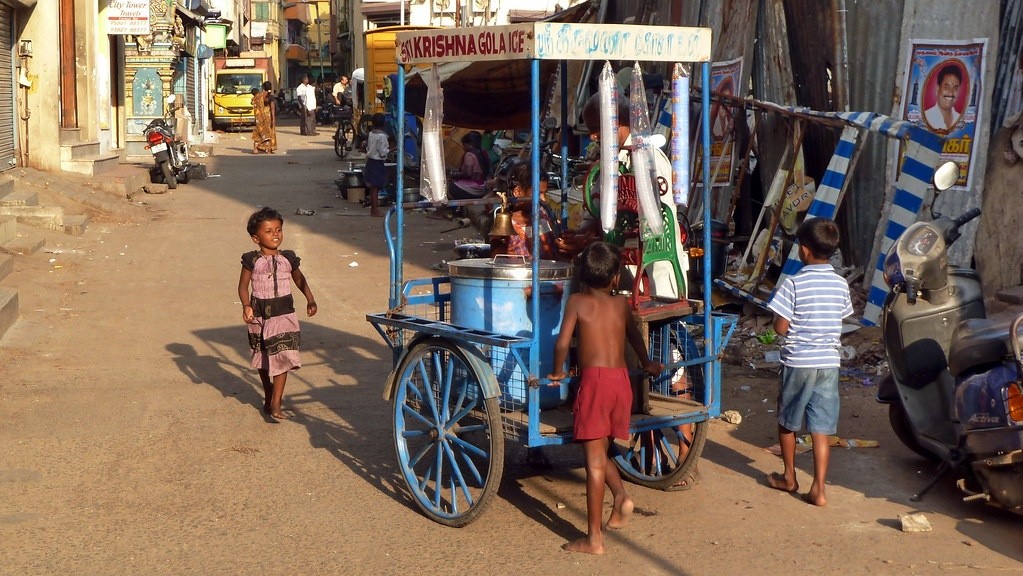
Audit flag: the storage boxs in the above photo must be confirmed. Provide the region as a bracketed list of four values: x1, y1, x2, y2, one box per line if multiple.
[347, 188, 365, 203]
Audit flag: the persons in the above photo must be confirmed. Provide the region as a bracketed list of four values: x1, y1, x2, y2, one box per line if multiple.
[274, 75, 352, 116]
[363, 113, 398, 217]
[237, 207, 318, 419]
[296, 74, 315, 136]
[766, 218, 855, 505]
[924, 66, 964, 131]
[547, 242, 665, 555]
[251, 88, 268, 116]
[433, 130, 496, 222]
[554, 91, 703, 492]
[488, 162, 560, 469]
[713, 84, 733, 137]
[306, 77, 320, 136]
[253, 81, 278, 155]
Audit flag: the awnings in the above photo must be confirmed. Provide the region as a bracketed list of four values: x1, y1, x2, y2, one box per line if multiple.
[382, 1, 596, 136]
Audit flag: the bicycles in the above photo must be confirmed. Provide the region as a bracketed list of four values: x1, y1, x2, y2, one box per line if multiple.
[328, 101, 356, 159]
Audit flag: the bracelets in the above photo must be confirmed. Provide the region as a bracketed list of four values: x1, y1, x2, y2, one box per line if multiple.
[243, 305, 253, 309]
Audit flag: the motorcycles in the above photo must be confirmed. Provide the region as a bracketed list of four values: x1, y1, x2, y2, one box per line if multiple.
[133, 93, 200, 189]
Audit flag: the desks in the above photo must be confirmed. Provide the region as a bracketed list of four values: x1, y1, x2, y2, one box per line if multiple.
[336, 169, 364, 200]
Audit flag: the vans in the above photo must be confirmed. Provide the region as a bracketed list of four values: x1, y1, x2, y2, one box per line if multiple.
[208, 68, 271, 130]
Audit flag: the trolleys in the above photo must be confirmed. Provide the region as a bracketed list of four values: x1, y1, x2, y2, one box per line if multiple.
[364, 19, 742, 531]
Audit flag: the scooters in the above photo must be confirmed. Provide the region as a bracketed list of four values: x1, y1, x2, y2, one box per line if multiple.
[874, 160, 1023, 528]
